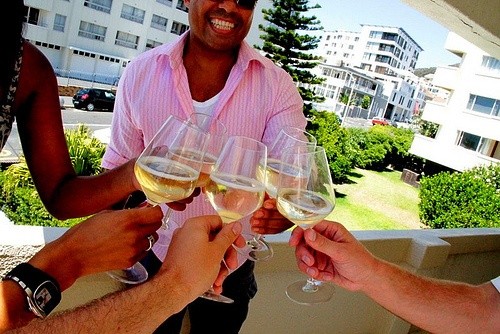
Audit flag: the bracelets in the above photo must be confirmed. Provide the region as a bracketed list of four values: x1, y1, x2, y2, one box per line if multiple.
[5, 262, 61, 320]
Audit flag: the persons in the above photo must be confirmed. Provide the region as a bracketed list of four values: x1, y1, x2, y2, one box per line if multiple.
[0, 216, 246, 334]
[100, 0, 317, 332]
[0, 204, 163, 334]
[0, 0, 201, 220]
[289, 220, 500, 334]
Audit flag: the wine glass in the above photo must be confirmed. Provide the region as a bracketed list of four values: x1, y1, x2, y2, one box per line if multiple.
[198, 136, 268, 303]
[231, 127, 318, 262]
[275, 145, 336, 304]
[105, 115, 210, 283]
[155, 112, 228, 245]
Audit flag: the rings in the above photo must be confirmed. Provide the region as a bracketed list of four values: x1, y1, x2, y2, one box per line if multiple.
[146, 235, 154, 251]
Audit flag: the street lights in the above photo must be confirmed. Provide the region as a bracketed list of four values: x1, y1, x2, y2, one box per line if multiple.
[338, 69, 357, 128]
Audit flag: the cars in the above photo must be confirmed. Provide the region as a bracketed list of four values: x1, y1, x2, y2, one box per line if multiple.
[72, 88, 116, 112]
[371, 117, 389, 126]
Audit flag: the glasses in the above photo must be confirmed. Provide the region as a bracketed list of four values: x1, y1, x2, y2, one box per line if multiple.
[236, 0, 259, 10]
